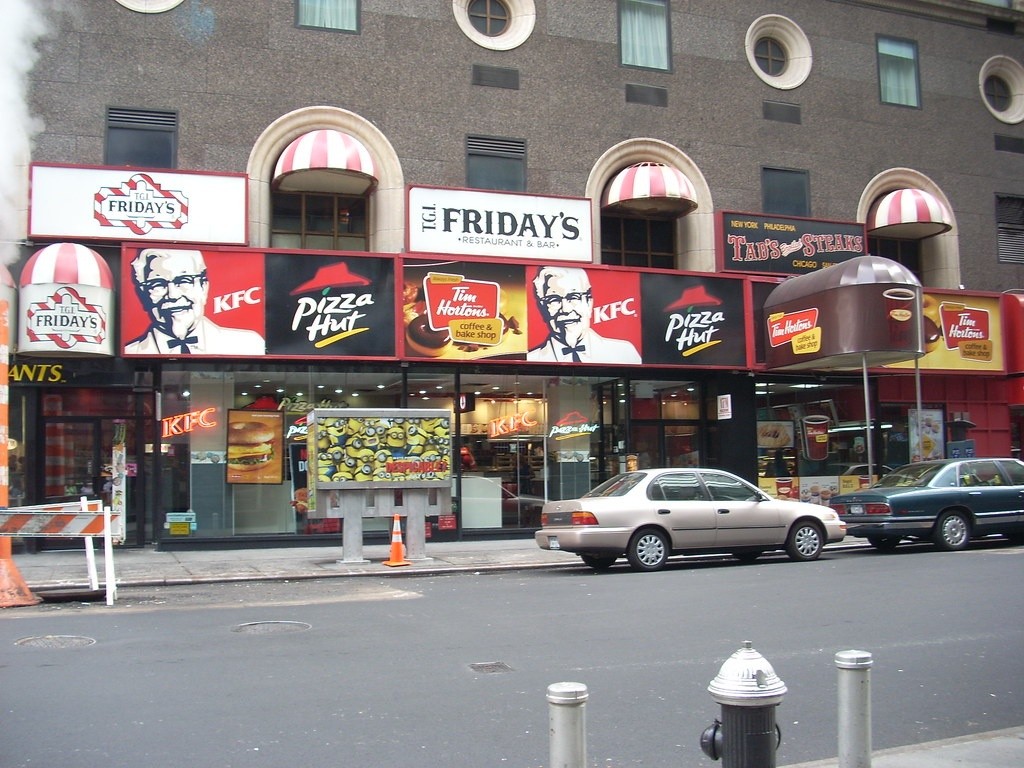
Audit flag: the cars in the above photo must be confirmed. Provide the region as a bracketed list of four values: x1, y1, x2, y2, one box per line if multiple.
[452, 475, 552, 527]
[829, 463, 916, 482]
[535, 468, 847, 572]
[829, 458, 1024, 551]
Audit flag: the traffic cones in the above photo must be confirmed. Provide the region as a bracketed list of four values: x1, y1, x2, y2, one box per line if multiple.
[382, 514, 411, 567]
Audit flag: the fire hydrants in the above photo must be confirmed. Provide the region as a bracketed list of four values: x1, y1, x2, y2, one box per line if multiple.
[700, 641, 788, 768]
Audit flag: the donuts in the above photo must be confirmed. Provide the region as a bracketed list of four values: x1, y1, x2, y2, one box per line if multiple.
[922, 294, 943, 353]
[403, 280, 453, 357]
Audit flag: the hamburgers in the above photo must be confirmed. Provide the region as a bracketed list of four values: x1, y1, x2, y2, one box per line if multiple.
[226, 421, 275, 471]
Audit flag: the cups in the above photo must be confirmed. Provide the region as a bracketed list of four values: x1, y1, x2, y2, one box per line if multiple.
[859, 475, 869, 488]
[776, 479, 792, 497]
[882, 288, 916, 348]
[802, 414, 830, 461]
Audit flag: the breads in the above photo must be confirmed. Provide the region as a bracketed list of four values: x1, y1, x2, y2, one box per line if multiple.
[756, 424, 792, 447]
